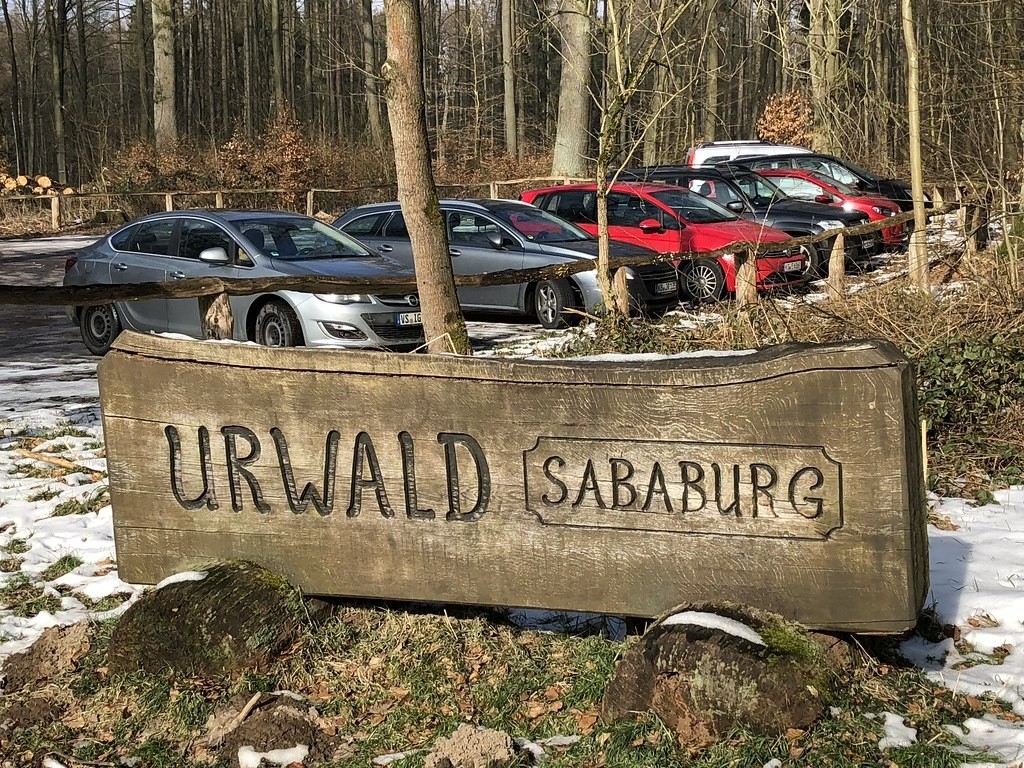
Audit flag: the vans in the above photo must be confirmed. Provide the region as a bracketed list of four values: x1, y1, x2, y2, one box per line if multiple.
[684, 140, 860, 203]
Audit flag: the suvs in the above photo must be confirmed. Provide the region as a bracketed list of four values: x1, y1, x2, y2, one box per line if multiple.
[605, 163, 880, 273]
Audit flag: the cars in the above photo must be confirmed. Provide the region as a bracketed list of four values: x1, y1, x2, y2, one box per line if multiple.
[510, 181, 808, 306]
[714, 154, 934, 227]
[62, 208, 427, 356]
[695, 168, 909, 249]
[313, 198, 679, 329]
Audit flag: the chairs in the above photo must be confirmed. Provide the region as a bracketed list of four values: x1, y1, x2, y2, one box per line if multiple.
[608, 197, 627, 225]
[778, 162, 789, 168]
[701, 183, 711, 196]
[243, 229, 274, 259]
[470, 216, 498, 247]
[450, 213, 469, 244]
[138, 233, 158, 253]
